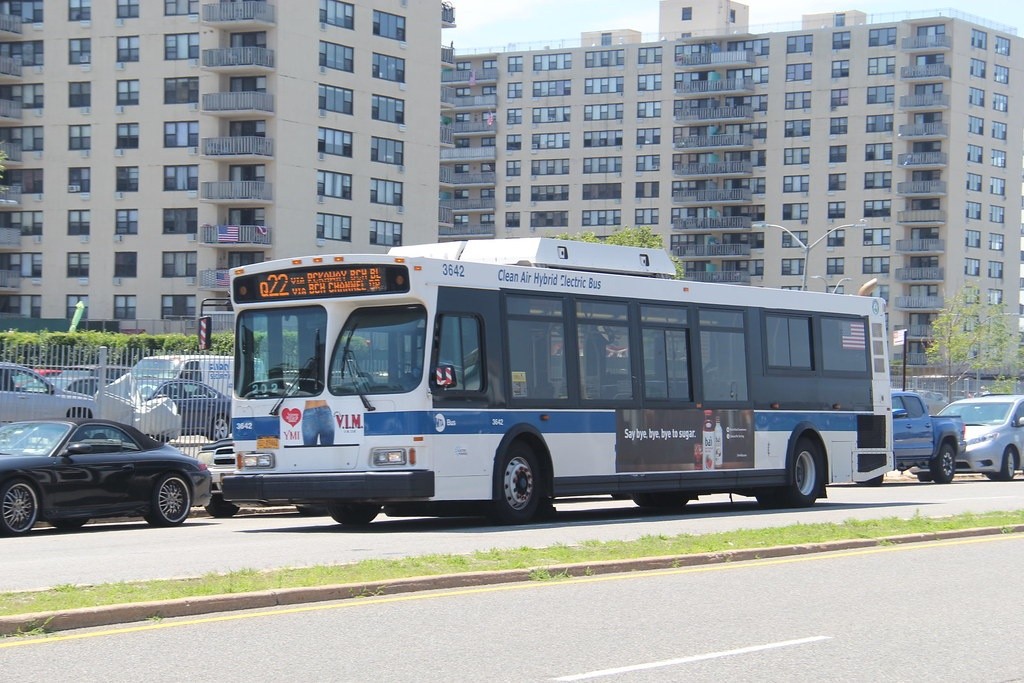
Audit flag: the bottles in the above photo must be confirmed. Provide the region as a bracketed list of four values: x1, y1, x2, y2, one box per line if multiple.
[703, 410, 716, 470]
[715, 416, 723, 466]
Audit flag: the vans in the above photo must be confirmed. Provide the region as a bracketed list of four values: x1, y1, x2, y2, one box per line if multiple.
[94, 356, 269, 399]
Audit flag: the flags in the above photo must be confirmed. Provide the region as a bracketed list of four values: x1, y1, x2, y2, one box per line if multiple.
[488, 112, 493, 126]
[217, 225, 239, 242]
[216, 273, 231, 286]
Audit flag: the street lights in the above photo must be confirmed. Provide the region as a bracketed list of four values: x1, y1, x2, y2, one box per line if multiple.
[752, 219, 866, 291]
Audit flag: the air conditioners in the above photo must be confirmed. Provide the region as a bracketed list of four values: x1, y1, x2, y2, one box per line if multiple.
[615, 65, 623, 204]
[112, 18, 123, 286]
[80, 20, 90, 285]
[399, 82, 407, 90]
[530, 71, 537, 232]
[882, 44, 895, 279]
[965, 46, 976, 280]
[317, 22, 326, 249]
[32, 23, 45, 286]
[398, 165, 408, 174]
[801, 52, 810, 225]
[400, 41, 408, 50]
[505, 71, 512, 233]
[187, 14, 197, 287]
[399, 124, 407, 132]
[634, 63, 644, 203]
[756, 82, 766, 281]
[671, 88, 677, 229]
[395, 206, 405, 214]
[826, 49, 838, 279]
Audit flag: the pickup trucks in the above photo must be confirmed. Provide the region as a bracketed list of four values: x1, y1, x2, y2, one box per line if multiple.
[853, 392, 967, 487]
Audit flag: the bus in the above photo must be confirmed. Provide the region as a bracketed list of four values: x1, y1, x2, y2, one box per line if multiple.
[197, 238, 897, 529]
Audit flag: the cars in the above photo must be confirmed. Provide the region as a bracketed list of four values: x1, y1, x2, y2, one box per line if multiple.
[129, 376, 233, 444]
[908, 394, 1024, 483]
[246, 369, 390, 399]
[20, 373, 116, 399]
[0, 418, 212, 538]
[0, 361, 95, 423]
[196, 436, 239, 520]
